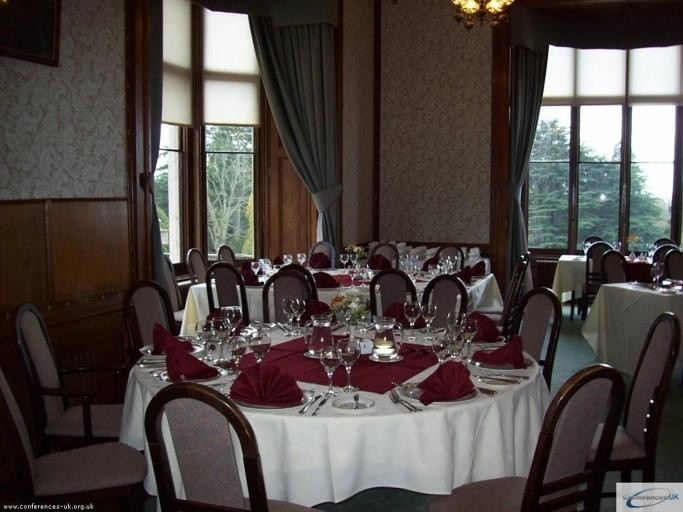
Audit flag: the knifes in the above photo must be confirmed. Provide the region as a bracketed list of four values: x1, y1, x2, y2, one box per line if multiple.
[311, 396, 327, 415]
[472, 373, 520, 385]
[353, 393, 359, 409]
[298, 394, 323, 414]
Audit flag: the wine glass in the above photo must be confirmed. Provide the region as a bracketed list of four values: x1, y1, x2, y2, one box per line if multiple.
[581, 241, 682, 293]
[192, 297, 477, 396]
[248, 241, 483, 287]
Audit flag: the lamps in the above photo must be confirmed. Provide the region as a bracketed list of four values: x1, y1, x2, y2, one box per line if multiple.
[446, 0, 519, 33]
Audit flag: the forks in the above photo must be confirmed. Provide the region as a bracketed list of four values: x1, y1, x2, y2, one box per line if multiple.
[388, 389, 423, 414]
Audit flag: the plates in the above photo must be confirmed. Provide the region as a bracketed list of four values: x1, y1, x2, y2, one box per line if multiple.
[138, 340, 204, 358]
[332, 397, 375, 410]
[159, 366, 226, 382]
[463, 336, 505, 345]
[477, 375, 516, 387]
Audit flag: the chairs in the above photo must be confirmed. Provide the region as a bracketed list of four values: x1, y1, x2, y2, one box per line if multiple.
[0, 236, 682, 512]
